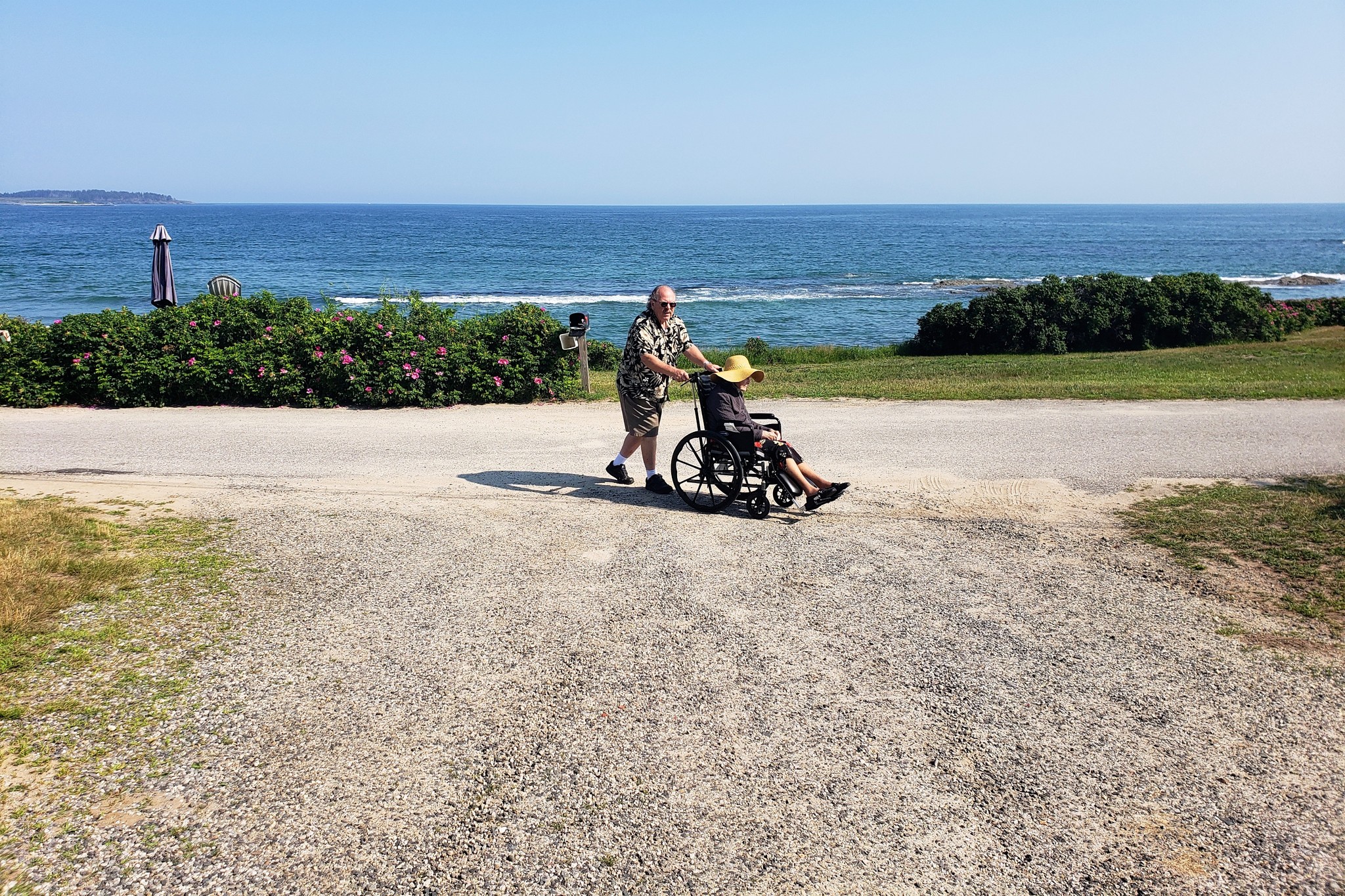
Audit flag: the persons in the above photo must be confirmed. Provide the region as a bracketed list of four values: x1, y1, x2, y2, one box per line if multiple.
[606, 285, 722, 494]
[706, 355, 850, 503]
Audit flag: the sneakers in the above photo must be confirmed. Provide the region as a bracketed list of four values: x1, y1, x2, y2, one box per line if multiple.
[806, 487, 837, 505]
[826, 483, 851, 492]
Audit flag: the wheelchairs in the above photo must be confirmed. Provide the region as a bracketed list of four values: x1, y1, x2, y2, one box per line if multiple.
[669, 367, 846, 519]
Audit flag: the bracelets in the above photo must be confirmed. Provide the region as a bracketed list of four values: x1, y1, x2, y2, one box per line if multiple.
[703, 360, 712, 370]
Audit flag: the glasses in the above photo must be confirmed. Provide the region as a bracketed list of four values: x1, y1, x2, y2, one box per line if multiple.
[653, 298, 676, 308]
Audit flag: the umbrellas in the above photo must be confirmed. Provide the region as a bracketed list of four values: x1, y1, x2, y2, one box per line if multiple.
[148, 224, 179, 310]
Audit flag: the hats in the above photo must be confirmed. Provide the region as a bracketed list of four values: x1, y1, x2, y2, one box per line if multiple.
[710, 355, 765, 383]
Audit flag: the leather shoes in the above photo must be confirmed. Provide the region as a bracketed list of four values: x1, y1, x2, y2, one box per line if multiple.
[606, 460, 635, 485]
[646, 473, 673, 494]
